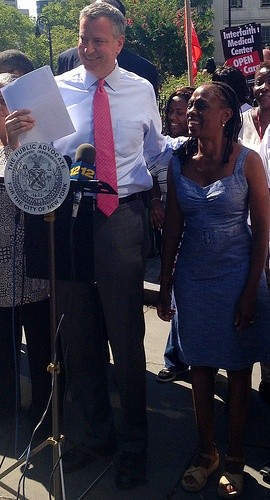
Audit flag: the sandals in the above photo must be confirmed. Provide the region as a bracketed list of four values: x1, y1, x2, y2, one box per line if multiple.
[218, 456, 246, 497]
[182, 451, 220, 491]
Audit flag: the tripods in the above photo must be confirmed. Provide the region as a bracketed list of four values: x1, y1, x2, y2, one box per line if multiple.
[0, 141, 150, 500]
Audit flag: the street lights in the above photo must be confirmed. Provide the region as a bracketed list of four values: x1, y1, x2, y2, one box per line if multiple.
[34, 15, 54, 76]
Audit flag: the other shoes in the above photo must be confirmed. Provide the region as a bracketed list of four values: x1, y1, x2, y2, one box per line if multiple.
[155, 364, 189, 381]
[62, 435, 106, 473]
[260, 466, 270, 477]
[116, 449, 148, 489]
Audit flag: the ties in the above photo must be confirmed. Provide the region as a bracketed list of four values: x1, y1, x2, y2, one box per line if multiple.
[92, 79, 119, 215]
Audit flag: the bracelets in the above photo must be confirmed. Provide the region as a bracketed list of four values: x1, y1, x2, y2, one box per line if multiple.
[151, 197, 160, 201]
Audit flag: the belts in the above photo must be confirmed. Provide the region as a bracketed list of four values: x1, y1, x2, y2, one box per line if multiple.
[85, 193, 139, 210]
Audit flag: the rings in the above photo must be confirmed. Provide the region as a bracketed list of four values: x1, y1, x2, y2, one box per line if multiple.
[248, 319, 255, 326]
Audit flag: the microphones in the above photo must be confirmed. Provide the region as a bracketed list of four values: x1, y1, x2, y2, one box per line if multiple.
[71, 143, 97, 224]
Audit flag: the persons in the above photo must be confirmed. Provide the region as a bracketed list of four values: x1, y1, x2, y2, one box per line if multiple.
[0, 72, 52, 434]
[3, 1, 195, 489]
[57, 0, 158, 99]
[0, 49, 45, 77]
[158, 41, 270, 476]
[156, 80, 270, 499]
